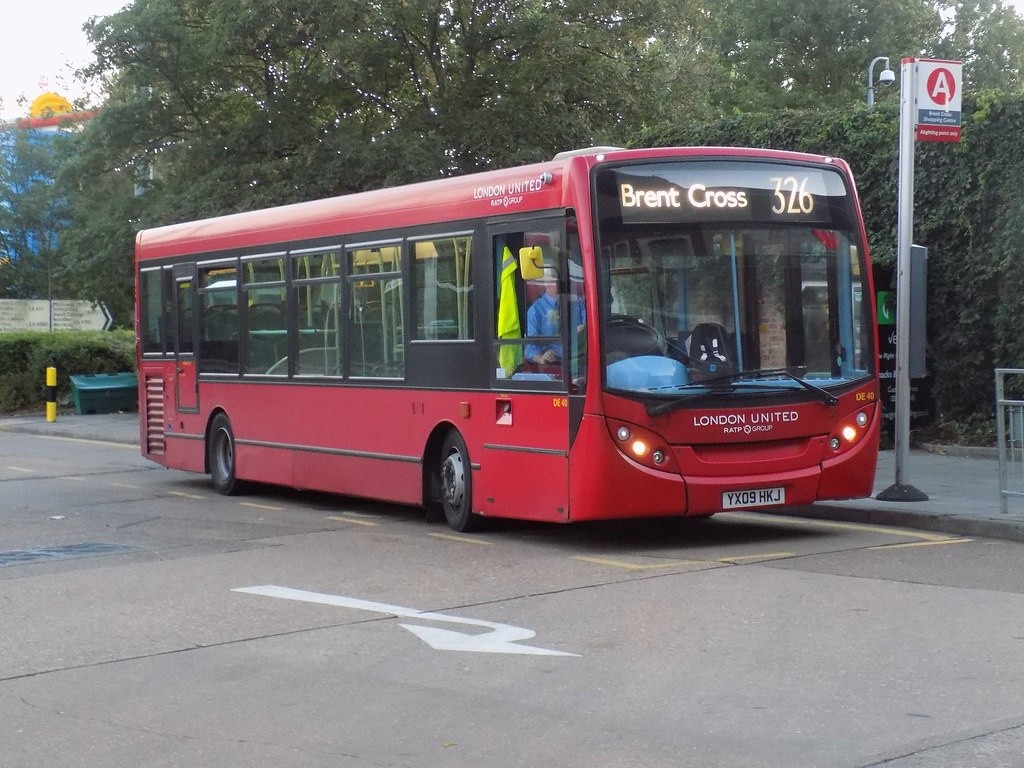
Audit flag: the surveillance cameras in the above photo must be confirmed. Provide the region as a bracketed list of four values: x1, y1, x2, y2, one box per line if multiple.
[880, 69, 895, 87]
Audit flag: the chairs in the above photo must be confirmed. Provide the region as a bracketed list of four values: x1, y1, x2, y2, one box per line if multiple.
[179, 302, 399, 368]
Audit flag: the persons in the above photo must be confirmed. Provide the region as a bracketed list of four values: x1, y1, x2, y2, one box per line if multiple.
[524, 271, 586, 380]
[802, 287, 830, 380]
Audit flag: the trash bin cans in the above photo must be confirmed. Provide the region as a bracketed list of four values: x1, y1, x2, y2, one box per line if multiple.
[69, 372, 138, 415]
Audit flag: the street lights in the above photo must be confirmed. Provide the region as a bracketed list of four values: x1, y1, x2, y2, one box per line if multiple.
[867, 56, 896, 110]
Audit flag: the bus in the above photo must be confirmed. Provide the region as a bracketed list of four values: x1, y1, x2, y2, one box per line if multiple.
[134, 146, 884, 534]
